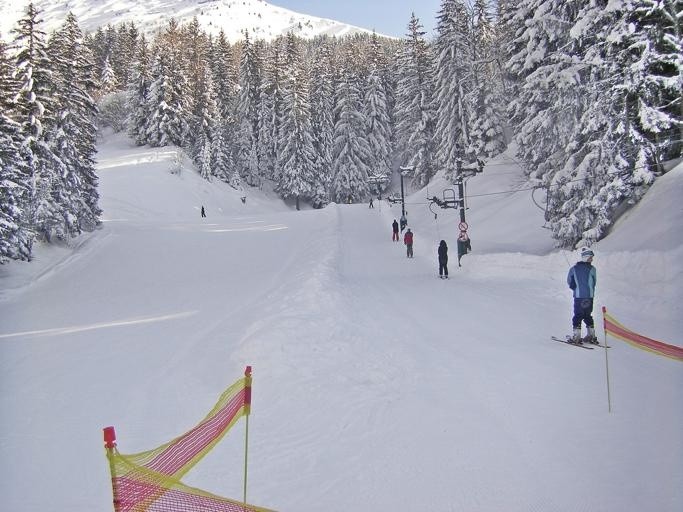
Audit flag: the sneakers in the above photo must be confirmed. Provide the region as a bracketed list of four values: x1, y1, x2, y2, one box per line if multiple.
[582, 335, 597, 343]
[567, 337, 583, 345]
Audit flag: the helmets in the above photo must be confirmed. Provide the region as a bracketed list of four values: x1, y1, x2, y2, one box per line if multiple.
[581, 251, 594, 263]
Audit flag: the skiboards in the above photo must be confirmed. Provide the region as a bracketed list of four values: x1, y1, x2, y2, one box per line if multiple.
[552, 335, 611, 350]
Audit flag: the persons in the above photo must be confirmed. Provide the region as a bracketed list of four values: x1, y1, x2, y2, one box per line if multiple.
[368, 198, 373, 207]
[565, 247, 597, 345]
[200, 206, 206, 218]
[437, 240, 448, 278]
[391, 219, 399, 242]
[403, 229, 413, 258]
